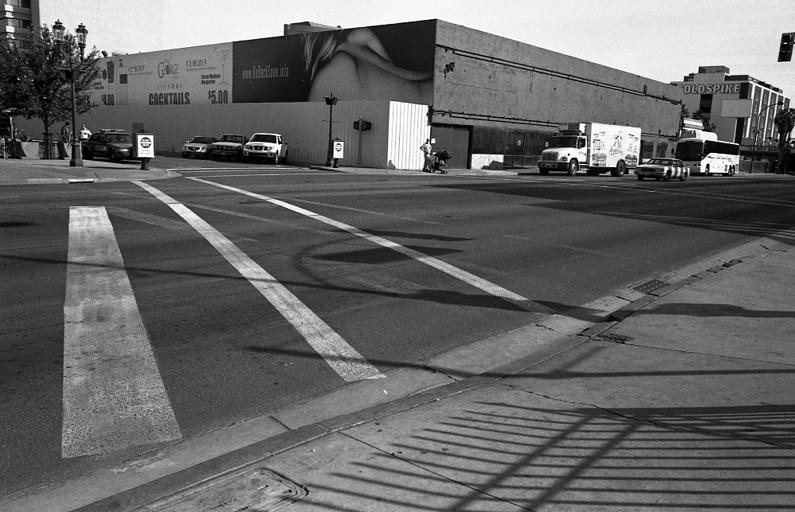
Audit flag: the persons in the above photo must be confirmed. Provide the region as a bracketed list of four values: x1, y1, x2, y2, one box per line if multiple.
[62, 121, 75, 144]
[300, 28, 433, 103]
[420, 138, 433, 174]
[76, 122, 92, 142]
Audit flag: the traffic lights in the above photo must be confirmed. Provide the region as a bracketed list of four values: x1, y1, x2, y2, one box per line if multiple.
[778, 32, 795, 61]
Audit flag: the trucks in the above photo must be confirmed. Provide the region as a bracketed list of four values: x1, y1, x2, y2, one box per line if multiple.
[538, 122, 641, 176]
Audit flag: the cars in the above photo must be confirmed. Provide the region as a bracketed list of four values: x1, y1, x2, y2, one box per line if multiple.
[82, 128, 136, 160]
[635, 158, 687, 182]
[180, 132, 288, 164]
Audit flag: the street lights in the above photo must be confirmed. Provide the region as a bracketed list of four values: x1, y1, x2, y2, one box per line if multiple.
[52, 19, 88, 167]
[749, 101, 783, 174]
[323, 91, 338, 167]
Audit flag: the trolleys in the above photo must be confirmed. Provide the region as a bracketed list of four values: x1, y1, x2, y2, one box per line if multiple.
[428, 151, 453, 174]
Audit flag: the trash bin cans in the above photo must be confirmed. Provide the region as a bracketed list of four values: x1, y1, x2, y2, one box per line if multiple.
[51, 139, 61, 159]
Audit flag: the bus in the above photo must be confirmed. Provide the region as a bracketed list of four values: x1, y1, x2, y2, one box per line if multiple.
[671, 138, 740, 176]
